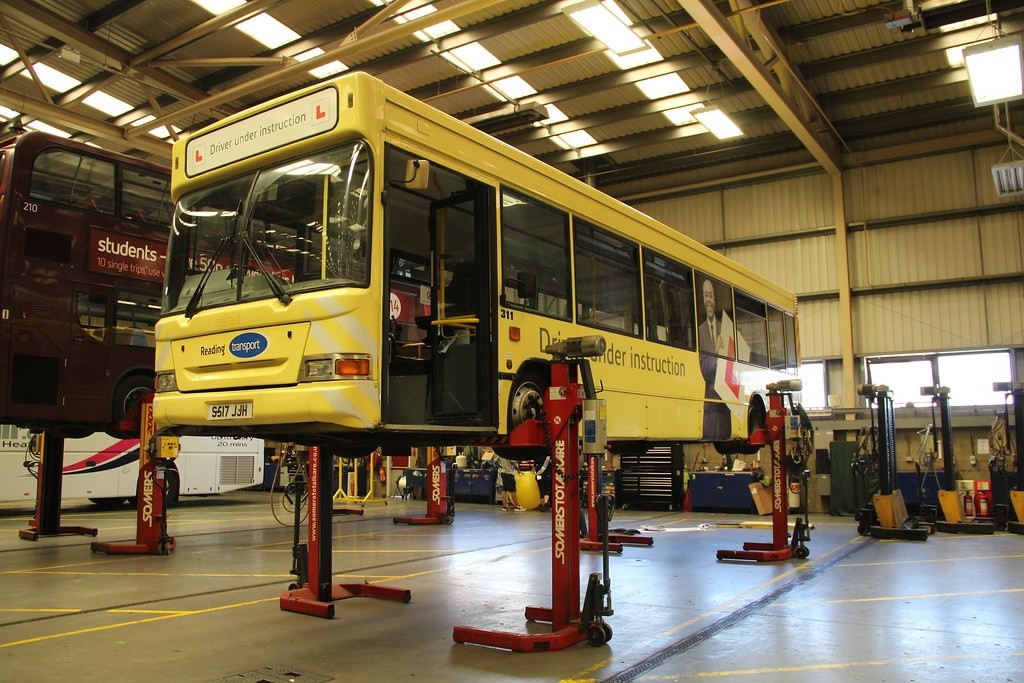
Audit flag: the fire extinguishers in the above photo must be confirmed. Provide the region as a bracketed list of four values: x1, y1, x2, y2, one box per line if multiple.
[977, 493, 988, 518]
[964, 490, 973, 516]
[379, 465, 386, 483]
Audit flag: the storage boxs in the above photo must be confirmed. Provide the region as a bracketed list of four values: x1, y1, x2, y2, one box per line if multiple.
[748, 483, 772, 516]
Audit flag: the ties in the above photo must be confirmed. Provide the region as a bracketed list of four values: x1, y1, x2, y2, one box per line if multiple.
[710, 325, 717, 350]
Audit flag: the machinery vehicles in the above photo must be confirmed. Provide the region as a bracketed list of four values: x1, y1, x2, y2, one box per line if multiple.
[853, 378, 937, 542]
[917, 384, 996, 536]
[985, 380, 1023, 534]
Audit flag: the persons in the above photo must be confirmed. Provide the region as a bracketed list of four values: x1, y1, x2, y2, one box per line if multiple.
[698, 280, 733, 439]
[493, 454, 552, 512]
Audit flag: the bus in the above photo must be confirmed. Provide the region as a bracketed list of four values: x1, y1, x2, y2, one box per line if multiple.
[0, 116, 435, 443]
[151, 68, 804, 457]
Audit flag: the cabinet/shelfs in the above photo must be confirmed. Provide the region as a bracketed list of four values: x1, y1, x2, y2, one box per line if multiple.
[895, 471, 947, 516]
[405, 468, 427, 500]
[690, 471, 761, 513]
[447, 468, 496, 505]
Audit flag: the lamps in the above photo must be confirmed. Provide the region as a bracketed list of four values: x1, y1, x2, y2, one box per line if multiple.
[991, 160, 1024, 197]
[962, 35, 1024, 107]
[690, 104, 746, 140]
